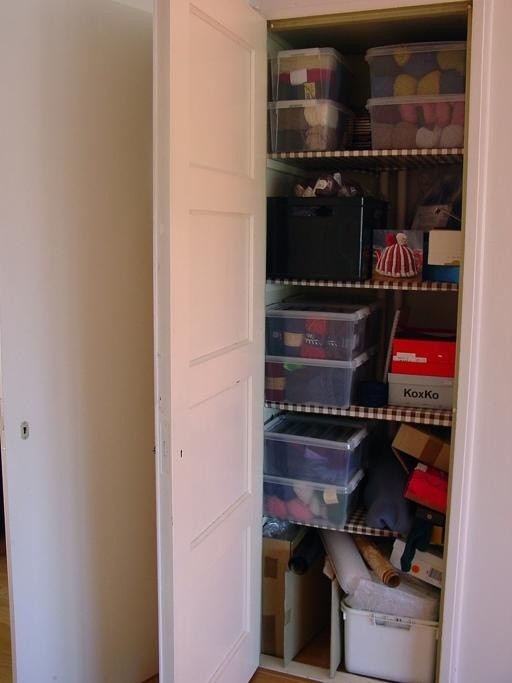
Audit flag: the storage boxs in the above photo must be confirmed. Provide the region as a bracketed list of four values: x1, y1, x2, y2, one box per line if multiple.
[262, 472, 363, 528]
[364, 44, 468, 98]
[339, 597, 440, 680]
[260, 521, 307, 662]
[387, 371, 452, 409]
[266, 194, 375, 283]
[269, 101, 350, 151]
[264, 412, 367, 486]
[265, 350, 371, 409]
[273, 45, 344, 98]
[390, 330, 455, 376]
[262, 301, 371, 360]
[368, 96, 464, 149]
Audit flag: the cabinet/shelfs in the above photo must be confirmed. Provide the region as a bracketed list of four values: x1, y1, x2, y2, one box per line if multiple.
[261, 150, 466, 677]
